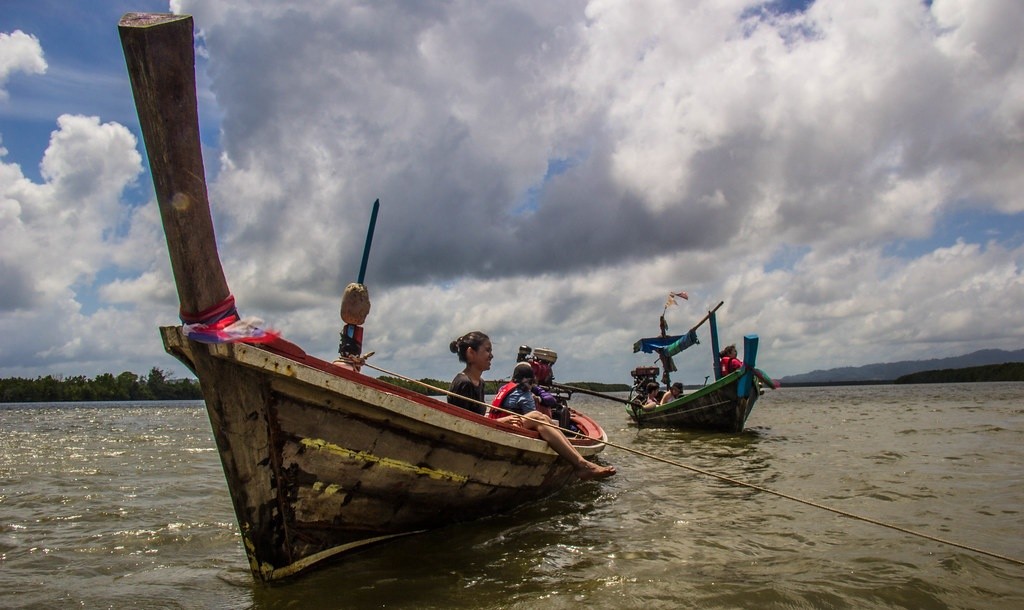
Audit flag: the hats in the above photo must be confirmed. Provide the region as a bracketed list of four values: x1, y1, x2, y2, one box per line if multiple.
[514, 362, 534, 378]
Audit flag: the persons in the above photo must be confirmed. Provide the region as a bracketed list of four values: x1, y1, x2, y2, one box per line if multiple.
[446, 330, 617, 480]
[645, 381, 660, 406]
[488, 363, 558, 420]
[717, 345, 743, 377]
[660, 382, 683, 405]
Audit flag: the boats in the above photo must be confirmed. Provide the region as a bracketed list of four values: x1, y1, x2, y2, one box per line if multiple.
[118, 13, 656, 585]
[625, 301, 765, 434]
